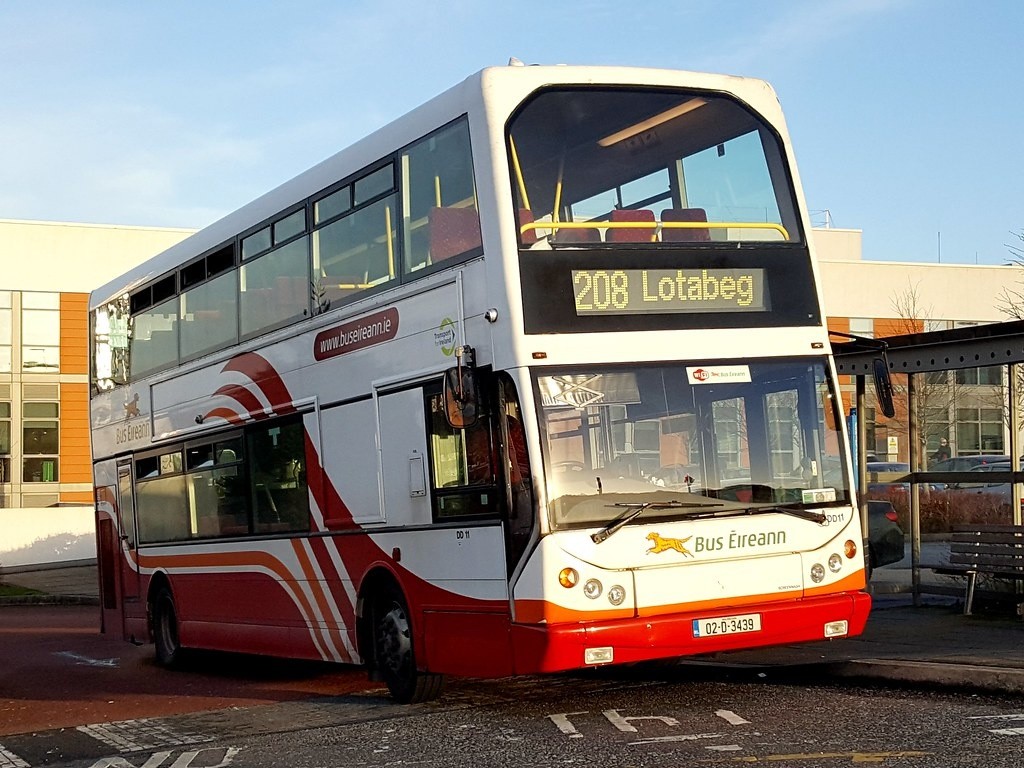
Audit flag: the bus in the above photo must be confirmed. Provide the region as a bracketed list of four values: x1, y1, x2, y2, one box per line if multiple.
[88, 58, 895, 703]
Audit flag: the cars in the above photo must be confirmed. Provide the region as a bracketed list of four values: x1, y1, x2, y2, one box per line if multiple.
[788, 454, 1024, 511]
[593, 450, 783, 486]
[857, 500, 905, 582]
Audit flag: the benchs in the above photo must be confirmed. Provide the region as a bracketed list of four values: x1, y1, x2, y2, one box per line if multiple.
[935, 524, 1024, 617]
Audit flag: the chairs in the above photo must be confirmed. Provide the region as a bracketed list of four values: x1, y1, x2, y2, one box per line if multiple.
[608, 209, 657, 252]
[127, 505, 298, 539]
[121, 279, 364, 376]
[662, 210, 710, 248]
[430, 209, 537, 270]
[554, 227, 605, 256]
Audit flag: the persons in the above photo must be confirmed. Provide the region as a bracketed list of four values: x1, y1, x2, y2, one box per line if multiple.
[458, 393, 531, 532]
[929, 436, 951, 462]
